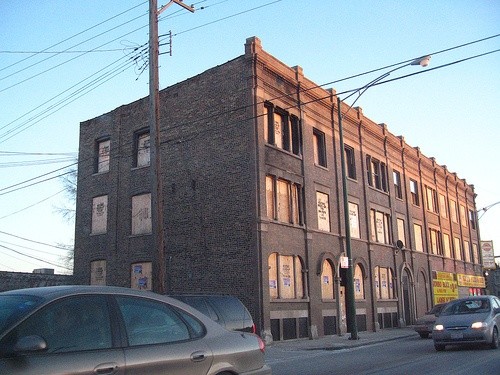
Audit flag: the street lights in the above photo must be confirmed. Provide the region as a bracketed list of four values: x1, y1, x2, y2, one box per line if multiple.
[335, 53, 430, 341]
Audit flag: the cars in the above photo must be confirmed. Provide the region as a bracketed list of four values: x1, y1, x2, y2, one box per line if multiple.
[434, 295, 500, 351]
[0, 285, 273, 375]
[414, 302, 447, 338]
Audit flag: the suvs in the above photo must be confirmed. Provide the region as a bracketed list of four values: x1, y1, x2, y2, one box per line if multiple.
[162, 295, 256, 336]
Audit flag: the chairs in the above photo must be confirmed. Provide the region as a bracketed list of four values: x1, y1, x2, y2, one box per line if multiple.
[458, 304, 470, 312]
[84, 318, 107, 347]
[127, 313, 155, 346]
